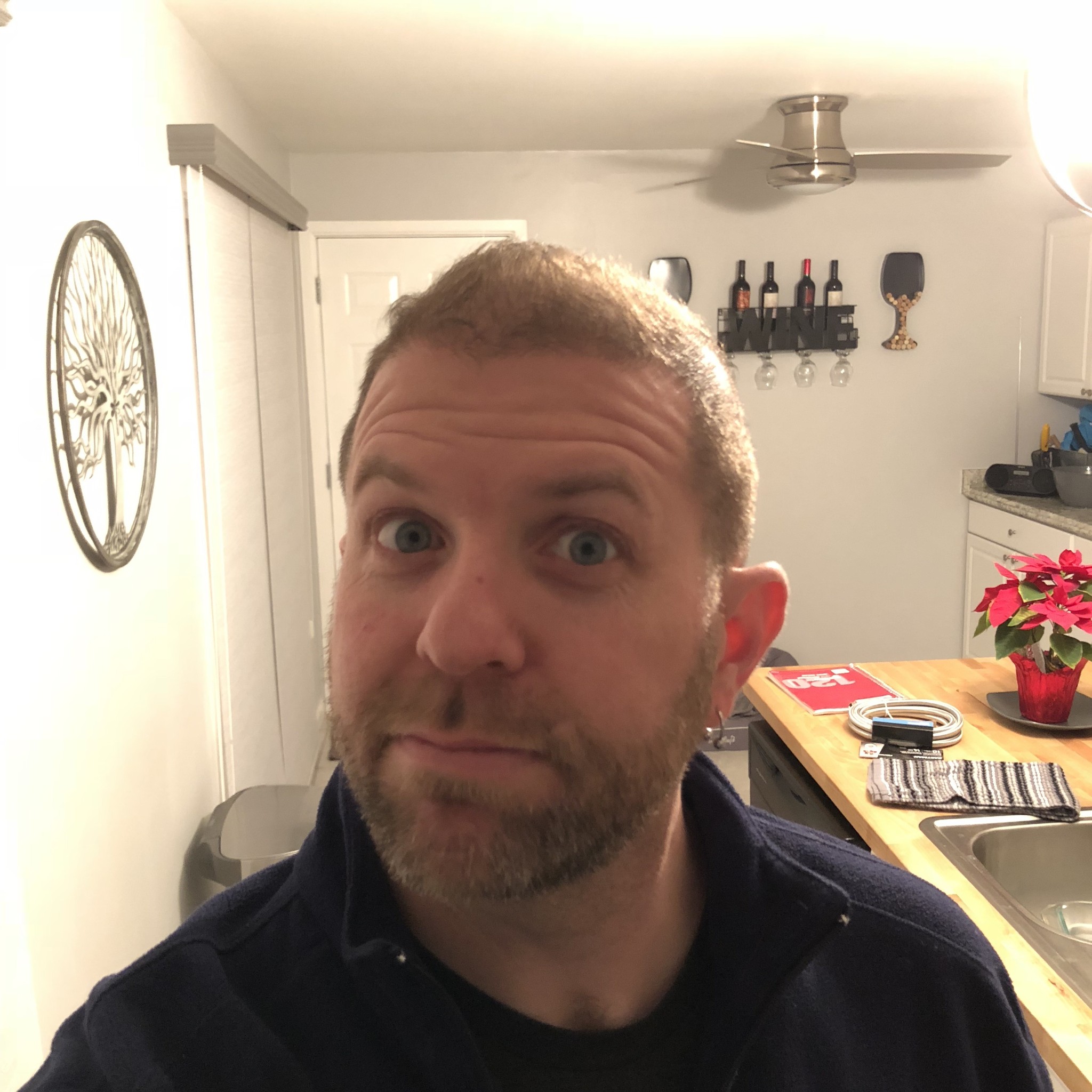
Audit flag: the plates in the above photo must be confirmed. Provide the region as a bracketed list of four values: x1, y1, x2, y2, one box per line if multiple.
[986, 689, 1092, 730]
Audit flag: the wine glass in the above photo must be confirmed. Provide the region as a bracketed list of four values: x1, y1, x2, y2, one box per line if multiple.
[794, 350, 817, 387]
[753, 352, 778, 390]
[723, 353, 738, 388]
[829, 348, 854, 386]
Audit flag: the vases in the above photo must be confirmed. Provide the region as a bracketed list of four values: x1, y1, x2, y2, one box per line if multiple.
[1007, 648, 1090, 724]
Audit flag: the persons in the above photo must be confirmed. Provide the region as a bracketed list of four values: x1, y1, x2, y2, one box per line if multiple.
[18, 236, 1054, 1092]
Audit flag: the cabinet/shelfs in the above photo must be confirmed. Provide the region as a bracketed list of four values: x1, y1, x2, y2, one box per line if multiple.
[960, 496, 1092, 658]
[1037, 213, 1092, 402]
[746, 720, 875, 854]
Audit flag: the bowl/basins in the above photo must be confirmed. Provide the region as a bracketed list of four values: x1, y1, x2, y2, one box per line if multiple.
[1049, 465, 1092, 508]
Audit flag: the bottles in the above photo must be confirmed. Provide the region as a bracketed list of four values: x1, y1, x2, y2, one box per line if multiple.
[824, 260, 843, 330]
[797, 259, 816, 329]
[732, 259, 750, 332]
[760, 261, 778, 331]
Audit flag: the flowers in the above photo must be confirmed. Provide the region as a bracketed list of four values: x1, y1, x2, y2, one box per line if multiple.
[970, 548, 1091, 676]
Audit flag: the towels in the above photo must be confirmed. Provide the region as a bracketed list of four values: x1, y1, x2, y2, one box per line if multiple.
[865, 754, 1083, 824]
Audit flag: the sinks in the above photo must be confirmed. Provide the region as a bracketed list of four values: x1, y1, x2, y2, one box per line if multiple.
[917, 804, 1092, 1014]
